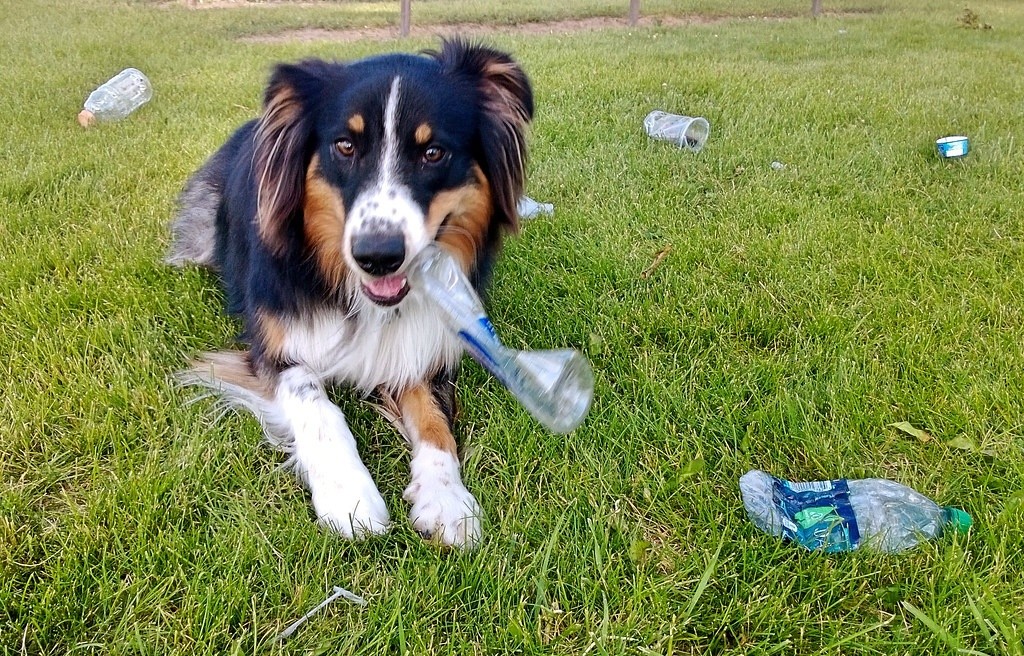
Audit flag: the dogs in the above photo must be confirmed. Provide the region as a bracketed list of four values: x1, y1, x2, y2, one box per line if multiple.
[168, 31, 535, 554]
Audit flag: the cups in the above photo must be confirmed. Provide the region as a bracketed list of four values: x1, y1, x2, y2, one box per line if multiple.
[640, 107, 710, 150]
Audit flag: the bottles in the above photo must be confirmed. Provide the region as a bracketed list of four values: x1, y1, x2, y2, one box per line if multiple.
[736, 466, 977, 561]
[78, 68, 154, 126]
[514, 192, 554, 222]
[411, 241, 595, 436]
